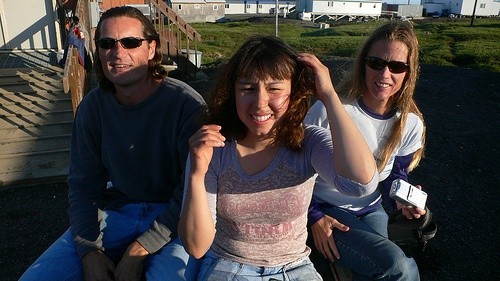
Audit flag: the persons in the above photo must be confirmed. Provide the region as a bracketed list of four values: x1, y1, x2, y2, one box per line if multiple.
[301, 22, 426, 281]
[178, 35, 378, 281]
[17, 5, 210, 281]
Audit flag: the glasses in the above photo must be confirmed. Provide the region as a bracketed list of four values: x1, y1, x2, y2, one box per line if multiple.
[97, 36, 149, 49]
[364, 55, 409, 74]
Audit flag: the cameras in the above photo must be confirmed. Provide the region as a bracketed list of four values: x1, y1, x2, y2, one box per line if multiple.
[389, 180, 428, 209]
